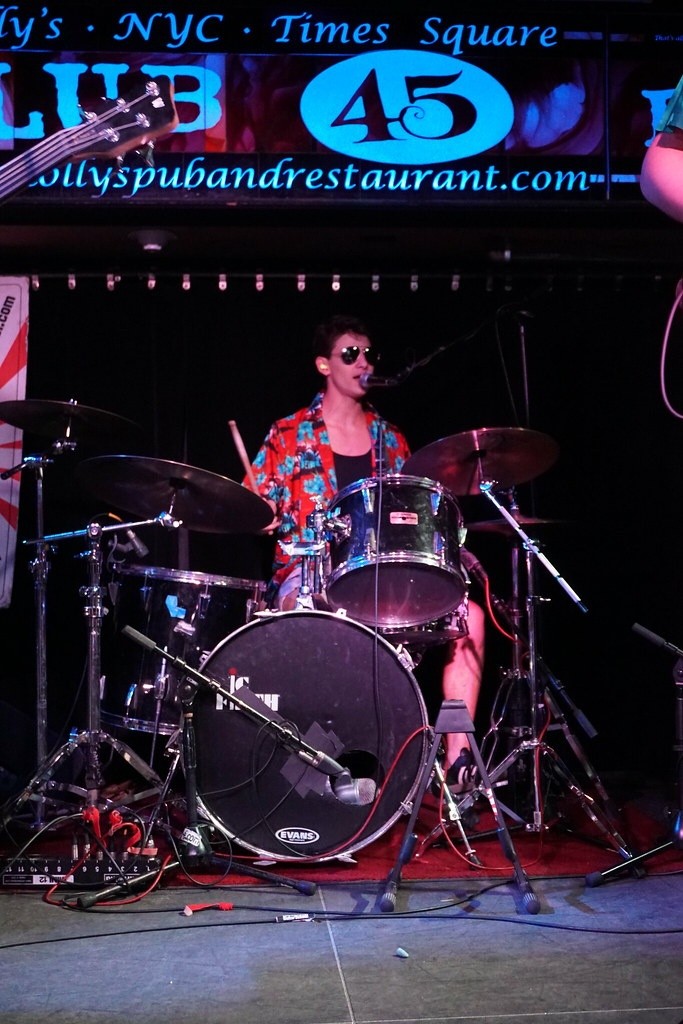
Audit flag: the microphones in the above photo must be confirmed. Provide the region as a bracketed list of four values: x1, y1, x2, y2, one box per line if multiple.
[331, 778, 379, 805]
[359, 373, 398, 388]
[109, 513, 150, 558]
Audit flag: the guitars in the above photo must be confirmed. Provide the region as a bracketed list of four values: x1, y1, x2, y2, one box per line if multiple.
[0, 76, 179, 200]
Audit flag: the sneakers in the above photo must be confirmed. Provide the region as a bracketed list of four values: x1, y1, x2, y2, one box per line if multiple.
[431, 748, 509, 799]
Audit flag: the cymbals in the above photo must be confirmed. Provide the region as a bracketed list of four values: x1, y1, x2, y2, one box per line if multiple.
[73, 453, 274, 539]
[398, 426, 555, 497]
[0, 390, 144, 443]
[468, 518, 559, 532]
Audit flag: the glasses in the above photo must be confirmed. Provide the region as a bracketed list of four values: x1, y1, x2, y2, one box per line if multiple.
[330, 346, 381, 365]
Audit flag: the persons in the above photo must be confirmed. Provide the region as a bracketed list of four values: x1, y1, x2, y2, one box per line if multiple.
[640, 76, 683, 222]
[241, 317, 486, 806]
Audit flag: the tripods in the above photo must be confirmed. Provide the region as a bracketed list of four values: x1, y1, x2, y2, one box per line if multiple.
[3, 413, 646, 909]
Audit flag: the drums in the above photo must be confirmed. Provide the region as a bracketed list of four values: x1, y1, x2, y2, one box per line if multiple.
[177, 608, 432, 866]
[315, 472, 469, 643]
[98, 560, 267, 739]
